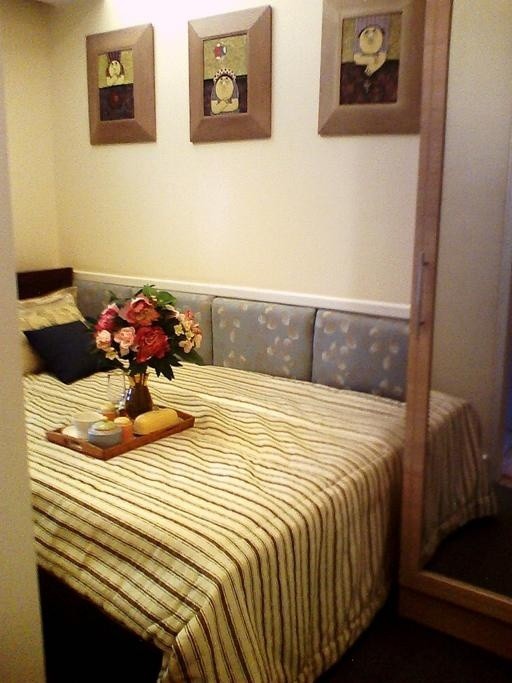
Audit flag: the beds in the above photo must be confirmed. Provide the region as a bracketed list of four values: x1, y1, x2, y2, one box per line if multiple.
[9, 266, 476, 683]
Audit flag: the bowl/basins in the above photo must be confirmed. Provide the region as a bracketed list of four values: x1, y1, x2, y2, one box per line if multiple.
[72, 410, 103, 433]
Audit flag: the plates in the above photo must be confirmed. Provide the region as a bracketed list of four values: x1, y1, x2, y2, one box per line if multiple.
[63, 426, 89, 441]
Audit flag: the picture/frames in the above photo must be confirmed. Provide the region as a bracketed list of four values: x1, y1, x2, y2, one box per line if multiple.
[85, 22, 158, 145]
[318, 0, 425, 135]
[188, 4, 272, 143]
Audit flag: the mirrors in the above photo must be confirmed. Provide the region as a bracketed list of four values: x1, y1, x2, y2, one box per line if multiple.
[393, 0, 512, 669]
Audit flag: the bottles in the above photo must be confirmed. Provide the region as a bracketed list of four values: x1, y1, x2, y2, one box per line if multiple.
[89, 416, 132, 450]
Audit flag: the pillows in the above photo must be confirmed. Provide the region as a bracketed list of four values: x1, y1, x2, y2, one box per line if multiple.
[15, 286, 120, 387]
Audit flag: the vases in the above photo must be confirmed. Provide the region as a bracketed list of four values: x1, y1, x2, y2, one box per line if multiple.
[124, 382, 154, 420]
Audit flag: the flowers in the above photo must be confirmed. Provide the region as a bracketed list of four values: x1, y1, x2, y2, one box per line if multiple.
[91, 281, 211, 386]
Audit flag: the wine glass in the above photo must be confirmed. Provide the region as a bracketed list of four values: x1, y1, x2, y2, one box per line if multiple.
[106, 374, 126, 416]
